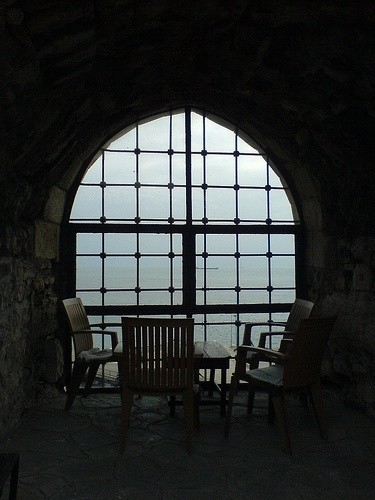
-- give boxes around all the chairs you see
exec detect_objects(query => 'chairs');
[237,298,317,425]
[54,297,138,416]
[112,315,208,462]
[227,315,337,456]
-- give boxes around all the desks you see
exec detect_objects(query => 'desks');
[112,340,231,443]
